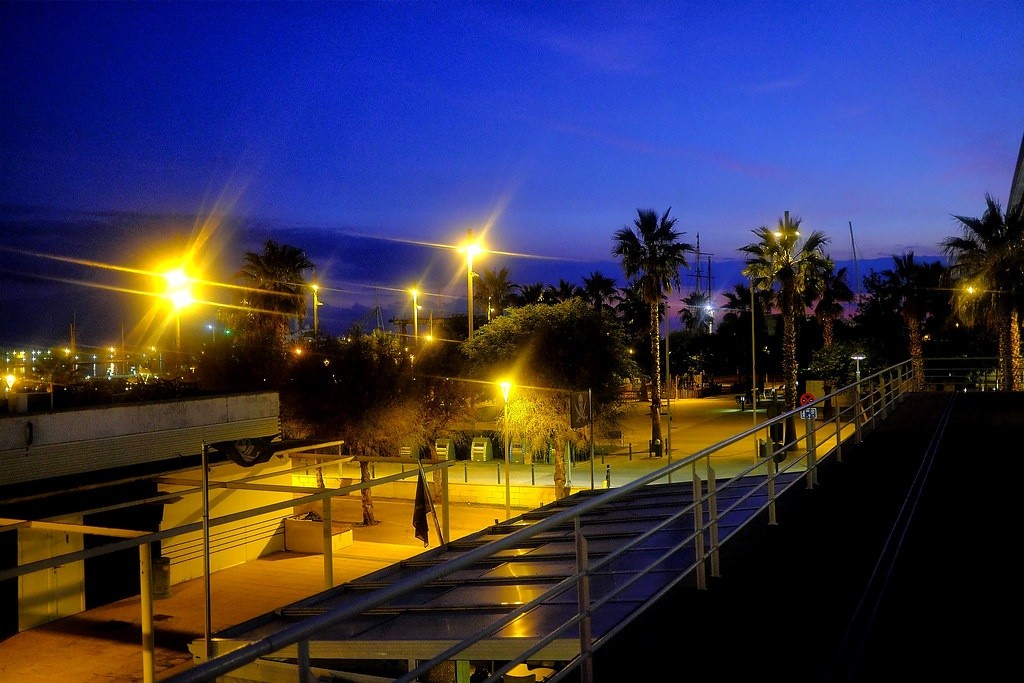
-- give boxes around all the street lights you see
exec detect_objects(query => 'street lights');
[166,270,190,376]
[309,278,322,336]
[851,350,864,445]
[408,287,419,352]
[457,228,487,345]
[495,381,514,518]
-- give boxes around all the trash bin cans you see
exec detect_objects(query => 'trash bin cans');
[400,445,420,457]
[435,438,456,460]
[759,439,767,457]
[510,438,531,464]
[549,438,570,465]
[470,437,493,462]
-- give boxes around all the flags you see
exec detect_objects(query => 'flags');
[413,472,433,549]
